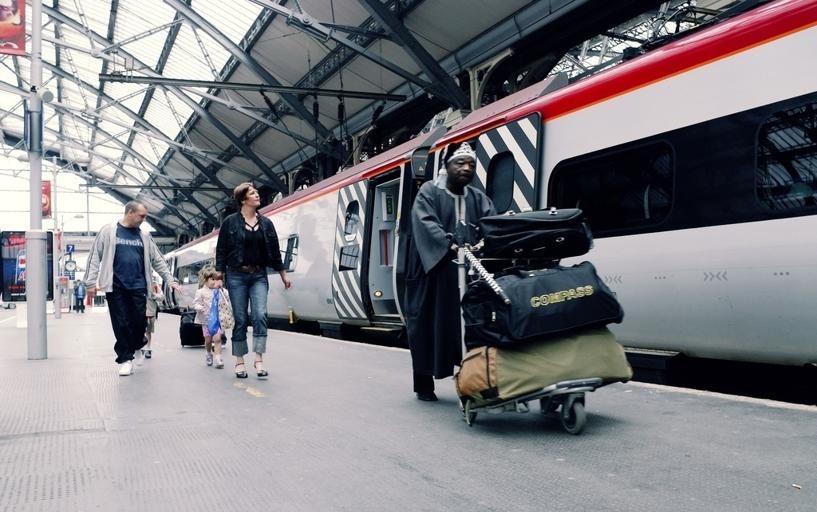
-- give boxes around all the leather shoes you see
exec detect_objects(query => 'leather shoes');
[235,363,247,378]
[254,361,268,376]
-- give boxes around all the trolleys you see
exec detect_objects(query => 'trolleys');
[450,244,602,435]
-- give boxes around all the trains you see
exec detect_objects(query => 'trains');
[149,1,813,370]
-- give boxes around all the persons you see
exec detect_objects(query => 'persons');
[191,265,234,369]
[399,142,500,403]
[139,270,166,359]
[72,278,104,314]
[82,199,185,379]
[210,182,293,379]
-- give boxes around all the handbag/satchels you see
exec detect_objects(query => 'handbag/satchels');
[457,326,633,411]
[218,288,235,329]
[460,262,623,350]
[479,209,589,260]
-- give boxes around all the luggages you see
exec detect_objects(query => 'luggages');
[180,310,205,347]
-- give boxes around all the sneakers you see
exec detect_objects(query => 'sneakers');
[118,360,134,376]
[135,343,151,366]
[207,354,224,369]
[416,392,438,401]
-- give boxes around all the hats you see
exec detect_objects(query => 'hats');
[444,140,477,164]
